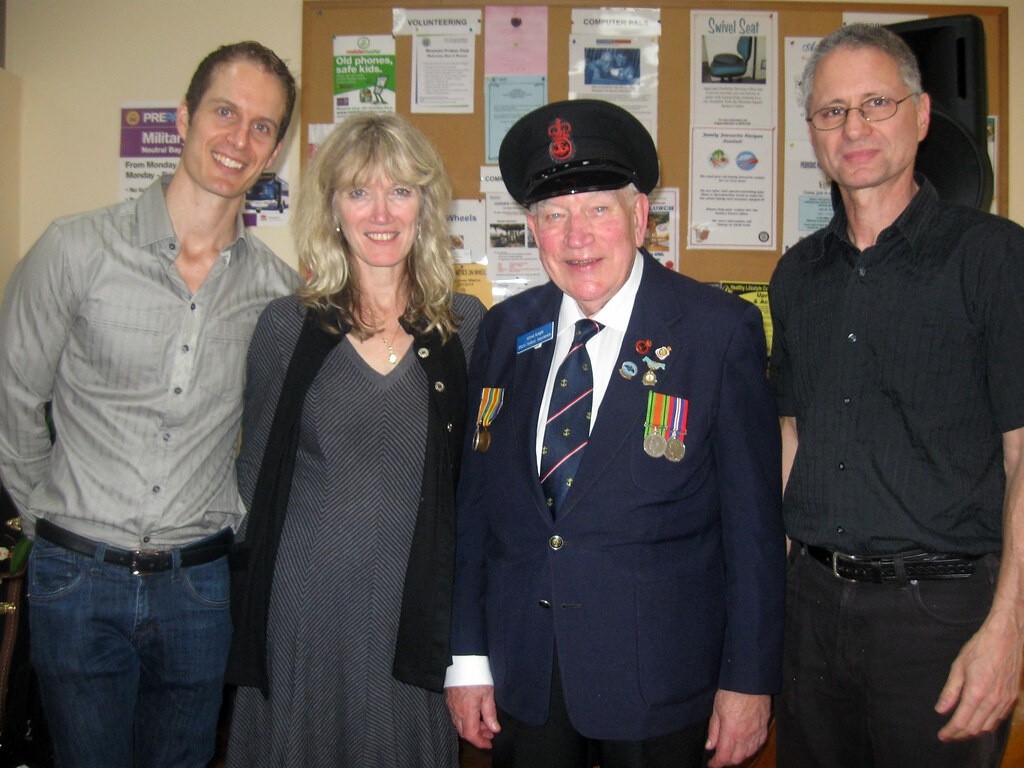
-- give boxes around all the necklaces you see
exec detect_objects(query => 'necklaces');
[379,324,402,365]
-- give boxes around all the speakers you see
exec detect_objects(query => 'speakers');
[831,14,994,217]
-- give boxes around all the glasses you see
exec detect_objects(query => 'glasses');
[806,92,921,131]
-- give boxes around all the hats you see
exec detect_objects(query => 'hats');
[498,98,660,212]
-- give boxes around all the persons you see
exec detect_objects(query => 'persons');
[0,39,309,768]
[221,112,489,768]
[441,99,787,768]
[769,22,1024,768]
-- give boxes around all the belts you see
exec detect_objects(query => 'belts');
[796,539,978,583]
[35,518,236,576]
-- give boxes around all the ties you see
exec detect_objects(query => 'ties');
[540,319,605,521]
